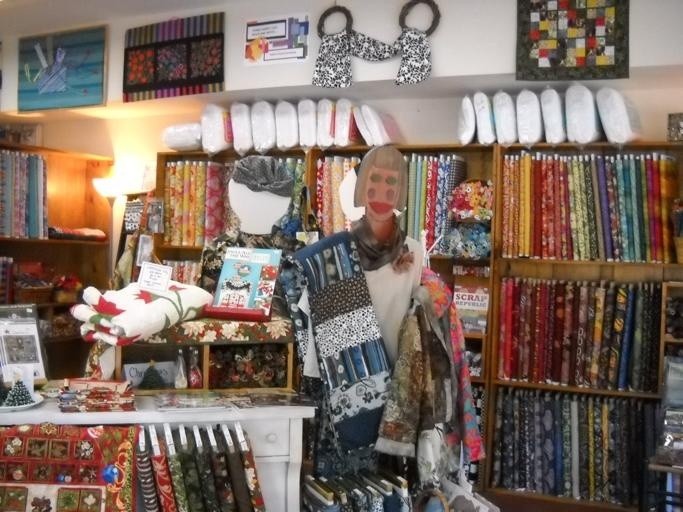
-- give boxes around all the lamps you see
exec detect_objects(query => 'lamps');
[89,177,129,295]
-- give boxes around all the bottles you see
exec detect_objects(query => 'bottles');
[189,347,203,388]
[175,348,188,389]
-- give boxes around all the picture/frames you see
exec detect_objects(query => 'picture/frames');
[514,0,629,81]
[15,23,109,115]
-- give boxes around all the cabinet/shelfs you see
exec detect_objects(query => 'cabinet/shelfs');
[0,142,115,375]
[483,146,668,510]
[304,150,495,487]
[155,149,303,312]
[0,388,318,512]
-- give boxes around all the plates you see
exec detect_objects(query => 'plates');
[0,393,45,413]
